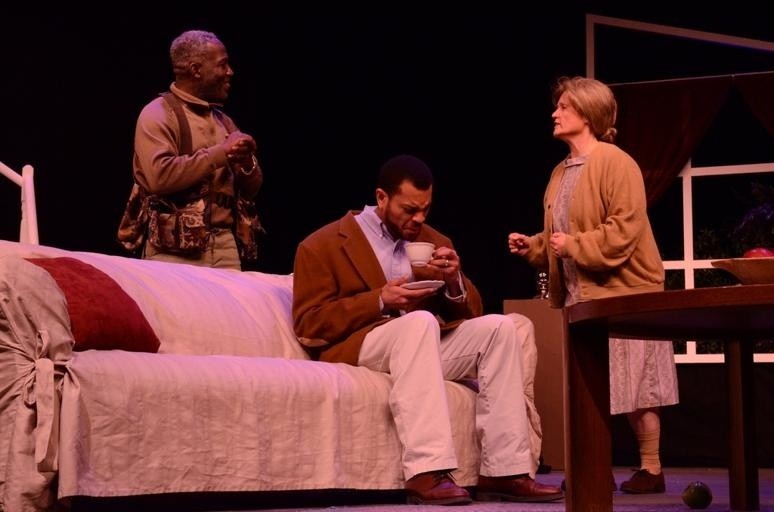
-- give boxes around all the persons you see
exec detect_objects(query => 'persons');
[508,76,679,496]
[116,31,266,273]
[291,153,565,507]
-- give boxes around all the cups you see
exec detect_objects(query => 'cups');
[404,242,437,268]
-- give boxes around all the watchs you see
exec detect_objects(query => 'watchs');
[242,153,256,174]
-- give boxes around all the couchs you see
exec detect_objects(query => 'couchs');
[0,239,542,511]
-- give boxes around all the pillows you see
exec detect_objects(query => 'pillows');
[26,256,161,354]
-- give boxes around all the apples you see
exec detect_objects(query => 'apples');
[743,248,774,259]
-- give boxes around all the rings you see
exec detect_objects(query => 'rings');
[444,259,450,268]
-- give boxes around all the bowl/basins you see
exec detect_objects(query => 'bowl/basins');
[711,258,774,286]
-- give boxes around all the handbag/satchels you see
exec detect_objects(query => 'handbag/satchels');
[236,190,267,263]
[145,181,211,255]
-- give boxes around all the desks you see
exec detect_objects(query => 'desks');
[564,283,773,512]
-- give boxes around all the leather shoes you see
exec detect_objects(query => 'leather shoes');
[403,470,474,507]
[561,471,617,492]
[476,473,564,504]
[621,469,666,495]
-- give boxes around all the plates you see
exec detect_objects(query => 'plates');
[396,280,446,293]
